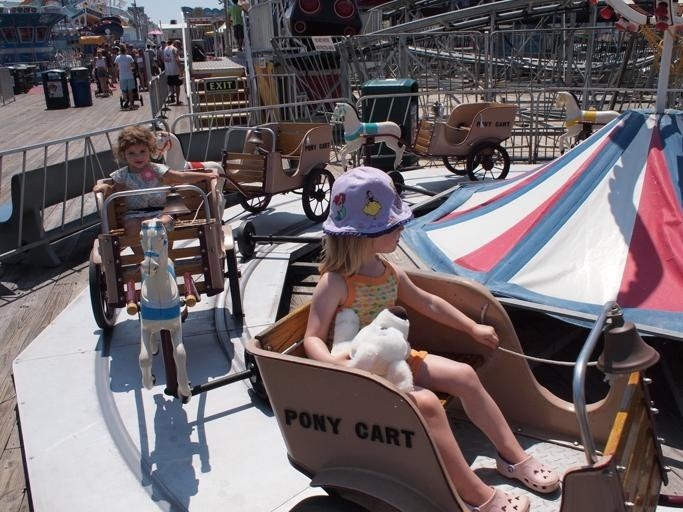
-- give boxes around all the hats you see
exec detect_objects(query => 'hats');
[321,166,414,239]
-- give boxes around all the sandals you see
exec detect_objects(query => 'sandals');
[466,486,530,512]
[496,452,560,493]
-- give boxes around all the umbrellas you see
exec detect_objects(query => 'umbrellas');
[147,29,164,35]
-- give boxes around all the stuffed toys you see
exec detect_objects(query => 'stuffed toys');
[332,306,414,391]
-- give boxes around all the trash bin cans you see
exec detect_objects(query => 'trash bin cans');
[360,78,418,170]
[67,67,92,108]
[41,69,71,110]
[5,64,39,95]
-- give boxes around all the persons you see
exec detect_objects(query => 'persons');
[227,0,248,51]
[93,126,219,260]
[302,168,560,511]
[71,36,204,111]
